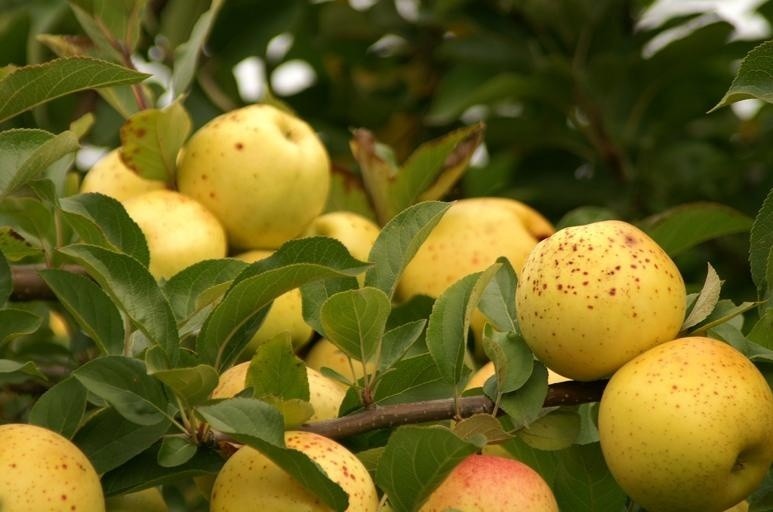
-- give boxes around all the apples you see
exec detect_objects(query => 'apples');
[0,103,773,512]
